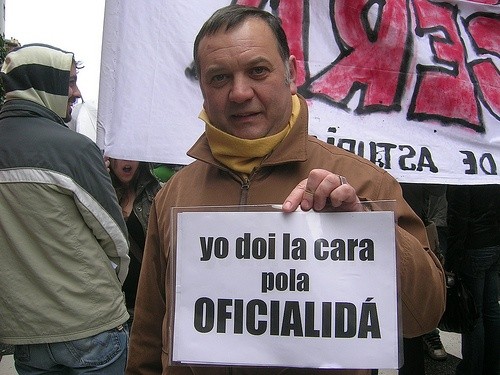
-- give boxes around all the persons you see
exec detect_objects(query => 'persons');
[1,41,128,375]
[103,155,163,334]
[398,183,500,375]
[125,5,447,372]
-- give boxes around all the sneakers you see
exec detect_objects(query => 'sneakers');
[423,330,448,360]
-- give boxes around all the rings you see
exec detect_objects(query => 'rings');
[338,174,347,185]
